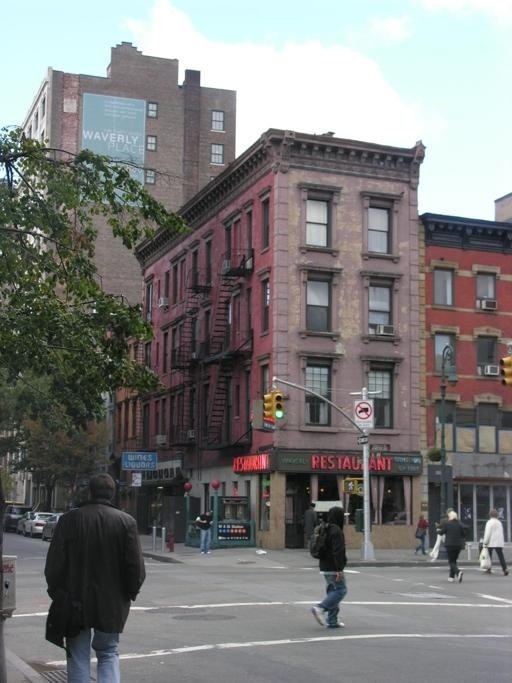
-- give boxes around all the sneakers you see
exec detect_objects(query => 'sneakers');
[200,550,212,555]
[457,570,463,583]
[447,577,455,583]
[484,568,493,575]
[312,604,328,627]
[330,622,346,628]
[504,569,509,576]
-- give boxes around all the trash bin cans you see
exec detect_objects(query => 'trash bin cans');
[468,541,480,561]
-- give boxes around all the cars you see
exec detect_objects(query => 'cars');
[386,510,408,523]
[3,499,64,543]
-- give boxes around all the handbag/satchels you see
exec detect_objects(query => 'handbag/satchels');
[414,526,426,538]
[42,576,89,654]
[479,548,493,568]
[195,520,206,528]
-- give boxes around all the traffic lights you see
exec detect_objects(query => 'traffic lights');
[262,393,273,419]
[274,392,283,418]
[345,478,356,492]
[498,354,512,387]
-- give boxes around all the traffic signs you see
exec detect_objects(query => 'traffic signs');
[357,436,370,444]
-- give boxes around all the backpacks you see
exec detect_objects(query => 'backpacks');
[309,521,332,560]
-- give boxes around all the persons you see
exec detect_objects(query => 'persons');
[435,507,454,528]
[45,472,147,681]
[414,513,430,555]
[435,510,465,583]
[197,507,214,553]
[482,508,509,576]
[302,502,319,548]
[311,505,348,628]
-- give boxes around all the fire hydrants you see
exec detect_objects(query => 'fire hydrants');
[166,526,176,552]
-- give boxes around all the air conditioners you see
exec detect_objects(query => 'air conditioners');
[376,323,397,337]
[481,298,498,311]
[220,257,234,274]
[187,429,196,439]
[483,364,500,376]
[158,297,170,308]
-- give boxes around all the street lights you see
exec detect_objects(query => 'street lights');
[431,344,458,558]
